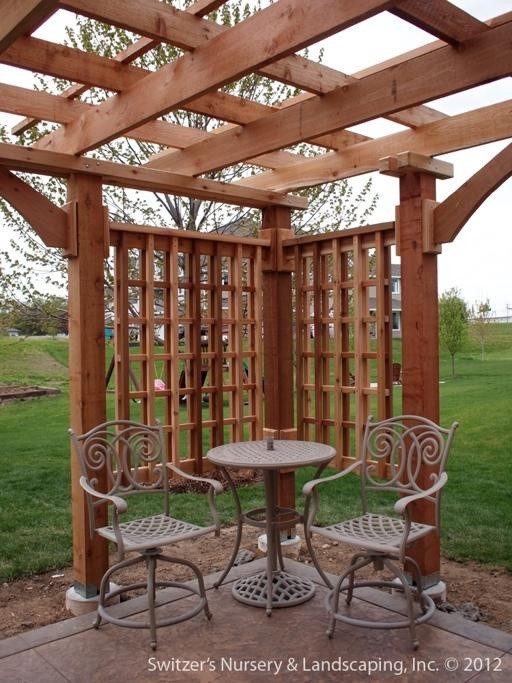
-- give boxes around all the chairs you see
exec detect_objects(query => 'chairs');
[301,415,459,653]
[67,420,226,651]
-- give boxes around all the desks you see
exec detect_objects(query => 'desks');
[207,439,336,617]
